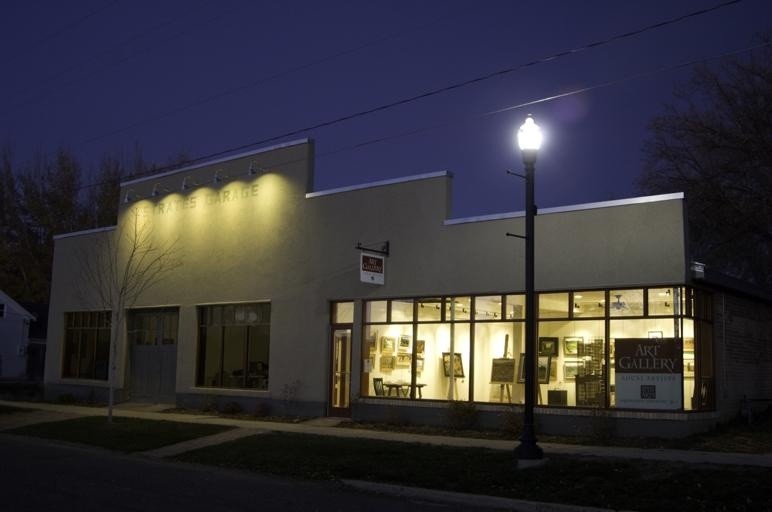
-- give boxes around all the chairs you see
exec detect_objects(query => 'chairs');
[373,377,399,397]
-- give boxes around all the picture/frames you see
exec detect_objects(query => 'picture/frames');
[517,335,615,384]
[441,351,463,377]
[491,358,515,383]
[369,326,426,373]
[648,330,695,380]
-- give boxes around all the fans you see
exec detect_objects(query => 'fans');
[593,295,636,312]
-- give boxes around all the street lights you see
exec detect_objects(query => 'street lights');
[506,111,548,463]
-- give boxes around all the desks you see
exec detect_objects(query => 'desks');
[402,383,428,399]
[383,383,403,397]
